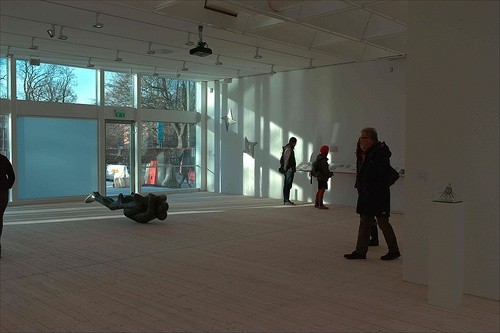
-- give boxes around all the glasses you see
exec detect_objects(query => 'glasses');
[360,136,367,140]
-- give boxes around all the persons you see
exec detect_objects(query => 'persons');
[0,154,16,236]
[344,128,401,260]
[178,147,192,188]
[354,138,379,246]
[314,145,334,210]
[278,137,297,205]
[85,191,169,223]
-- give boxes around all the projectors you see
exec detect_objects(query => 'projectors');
[189,41,212,57]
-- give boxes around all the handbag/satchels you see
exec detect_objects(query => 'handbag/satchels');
[389,165,400,187]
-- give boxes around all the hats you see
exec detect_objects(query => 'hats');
[320,145,329,154]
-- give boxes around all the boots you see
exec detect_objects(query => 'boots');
[314,199,329,210]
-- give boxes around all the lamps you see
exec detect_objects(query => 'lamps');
[5,46,13,58]
[29,14,277,77]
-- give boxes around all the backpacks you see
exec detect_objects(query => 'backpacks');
[311,157,327,177]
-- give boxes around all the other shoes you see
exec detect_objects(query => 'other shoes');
[366,239,380,247]
[284,201,295,206]
[380,252,401,260]
[343,250,367,260]
